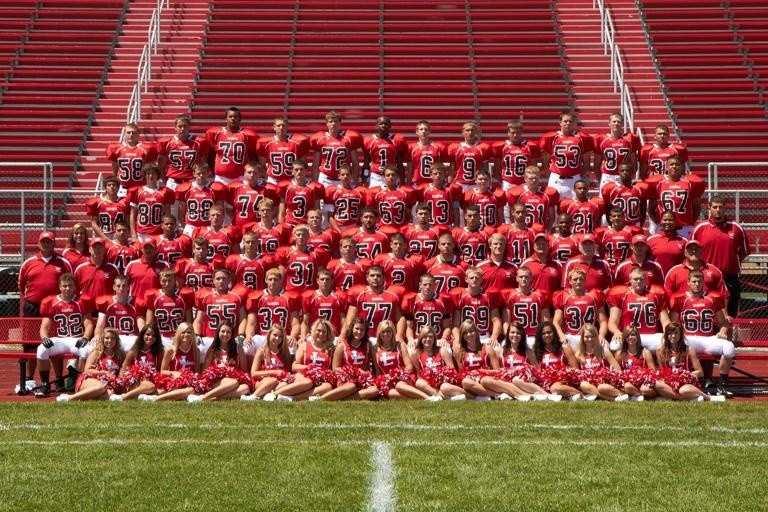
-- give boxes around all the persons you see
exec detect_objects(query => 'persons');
[15,107,750,401]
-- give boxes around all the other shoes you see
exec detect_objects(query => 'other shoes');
[425,394,645,403]
[688,379,733,402]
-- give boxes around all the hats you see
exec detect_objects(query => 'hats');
[39,231,56,242]
[534,233,703,250]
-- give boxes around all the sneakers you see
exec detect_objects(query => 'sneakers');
[308,394,322,402]
[263,390,276,401]
[241,394,260,401]
[54,378,66,392]
[56,393,69,404]
[138,393,157,403]
[277,394,294,402]
[34,381,51,396]
[110,394,125,402]
[186,394,203,403]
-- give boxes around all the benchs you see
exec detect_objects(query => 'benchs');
[0,353,768,396]
[0,0,768,259]
